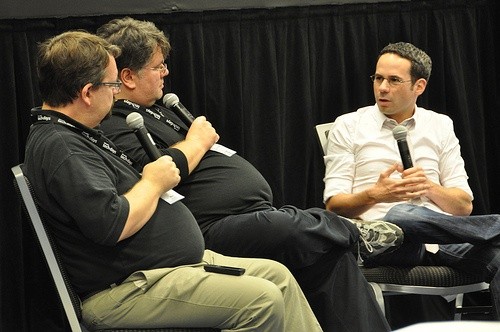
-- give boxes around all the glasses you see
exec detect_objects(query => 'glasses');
[120,62,167,74]
[81,79,122,94]
[370,74,415,88]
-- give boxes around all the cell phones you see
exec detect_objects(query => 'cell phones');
[204,265,246,276]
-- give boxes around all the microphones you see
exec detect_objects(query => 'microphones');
[162,93,195,129]
[393,125,413,171]
[127,112,161,161]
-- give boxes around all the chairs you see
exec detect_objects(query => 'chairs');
[12,164,230,332]
[315,123,493,321]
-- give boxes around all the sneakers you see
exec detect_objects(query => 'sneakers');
[338,215,404,266]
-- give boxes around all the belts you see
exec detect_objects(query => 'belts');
[80,280,122,301]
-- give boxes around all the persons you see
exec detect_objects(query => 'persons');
[96,17,405,332]
[324,42,500,317]
[24,32,323,332]
